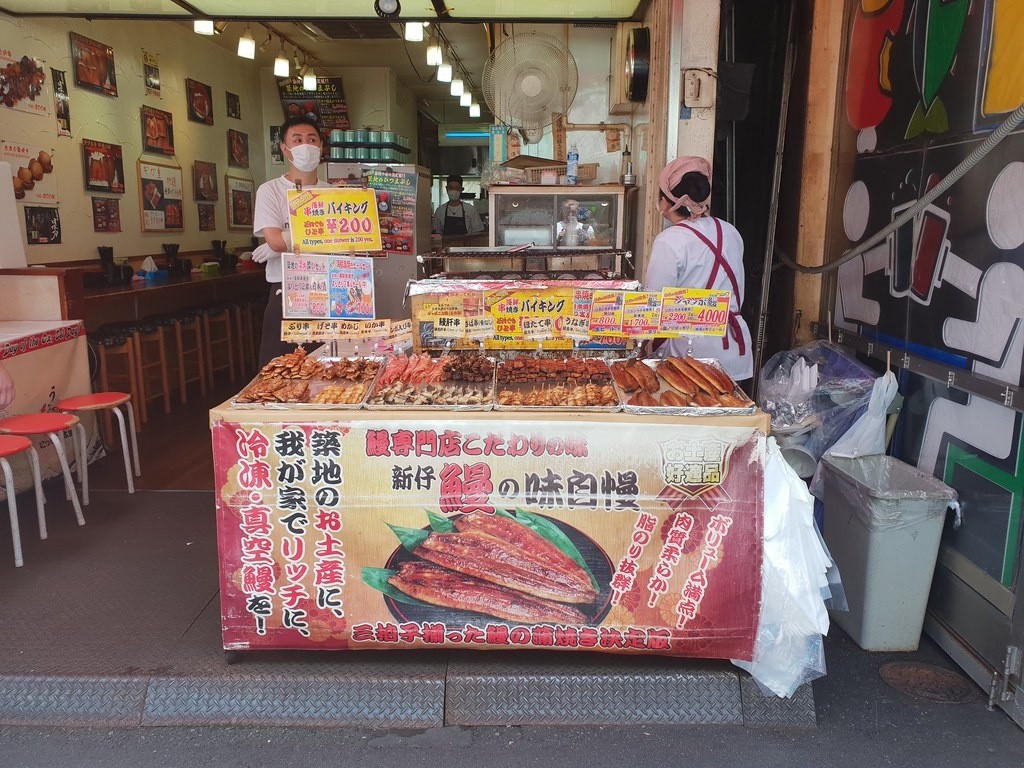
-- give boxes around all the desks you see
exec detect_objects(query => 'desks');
[56,392,142,501]
[83,265,266,302]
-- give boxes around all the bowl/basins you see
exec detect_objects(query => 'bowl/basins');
[780,445,817,478]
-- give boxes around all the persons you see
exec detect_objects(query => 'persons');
[636,155,753,397]
[552,200,596,245]
[432,174,486,235]
[252,117,339,371]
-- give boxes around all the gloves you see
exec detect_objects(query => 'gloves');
[251,242,281,264]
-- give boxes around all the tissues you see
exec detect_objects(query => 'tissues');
[201,262,220,272]
[140,255,168,279]
[239,251,255,267]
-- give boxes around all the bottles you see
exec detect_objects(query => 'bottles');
[566,143,579,186]
[621,144,632,174]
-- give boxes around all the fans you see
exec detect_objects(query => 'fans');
[480,30,579,145]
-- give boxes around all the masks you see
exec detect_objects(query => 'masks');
[280,144,322,173]
[448,190,460,200]
[656,197,669,215]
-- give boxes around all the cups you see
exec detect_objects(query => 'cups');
[102,263,114,277]
[114,264,134,282]
[176,259,194,273]
[167,257,177,265]
[224,255,238,267]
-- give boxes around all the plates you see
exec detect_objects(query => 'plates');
[384,509,616,630]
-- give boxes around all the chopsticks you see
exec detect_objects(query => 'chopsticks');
[212,240,226,252]
[97,246,113,263]
[162,244,179,256]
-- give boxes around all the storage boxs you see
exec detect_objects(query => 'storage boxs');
[500,228,551,245]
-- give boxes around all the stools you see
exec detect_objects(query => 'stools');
[0,435,48,568]
[85,298,264,447]
[0,412,89,526]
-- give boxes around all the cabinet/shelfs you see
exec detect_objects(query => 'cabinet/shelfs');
[606,21,642,115]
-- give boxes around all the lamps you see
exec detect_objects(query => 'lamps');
[237,21,255,60]
[273,37,289,77]
[258,28,272,53]
[214,20,229,34]
[297,52,317,91]
[436,43,452,83]
[293,46,302,70]
[193,20,213,36]
[405,22,424,42]
[450,60,481,118]
[426,23,442,66]
[373,0,401,18]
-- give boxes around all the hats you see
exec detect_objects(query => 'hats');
[446,174,463,185]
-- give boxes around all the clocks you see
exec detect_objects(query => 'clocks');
[624,26,649,102]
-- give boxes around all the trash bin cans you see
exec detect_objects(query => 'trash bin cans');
[820,454,957,651]
